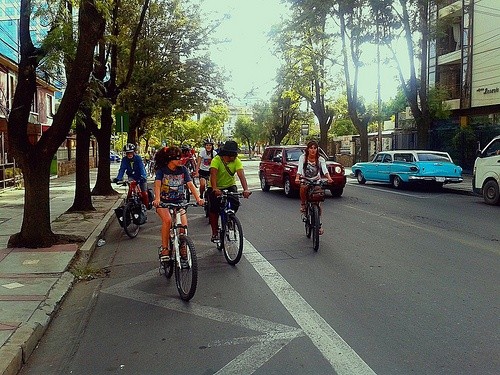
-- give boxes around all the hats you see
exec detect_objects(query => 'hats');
[222,140,241,153]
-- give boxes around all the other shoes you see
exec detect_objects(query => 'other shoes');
[318,227,324,235]
[300,212,307,221]
[179,245,187,259]
[161,247,170,262]
[227,222,234,231]
[210,235,218,241]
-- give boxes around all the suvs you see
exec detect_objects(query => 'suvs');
[258,144,347,198]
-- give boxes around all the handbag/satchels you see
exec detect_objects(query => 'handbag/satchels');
[309,184,325,203]
[114,205,131,227]
[132,203,147,225]
[147,188,154,210]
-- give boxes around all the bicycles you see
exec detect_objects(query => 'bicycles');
[153,200,208,301]
[144,158,155,178]
[300,176,332,251]
[212,192,252,264]
[111,178,149,237]
[184,173,196,208]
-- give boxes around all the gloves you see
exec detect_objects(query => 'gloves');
[140,177,146,183]
[113,178,119,183]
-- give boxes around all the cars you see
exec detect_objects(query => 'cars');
[110,151,120,163]
[351,150,465,190]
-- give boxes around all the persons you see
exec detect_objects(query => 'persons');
[152,145,205,269]
[195,141,218,203]
[295,140,334,235]
[113,143,149,209]
[207,141,251,242]
[143,141,221,178]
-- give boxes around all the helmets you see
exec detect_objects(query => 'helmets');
[204,138,214,144]
[123,143,135,151]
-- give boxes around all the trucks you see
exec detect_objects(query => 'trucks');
[472,135,500,205]
